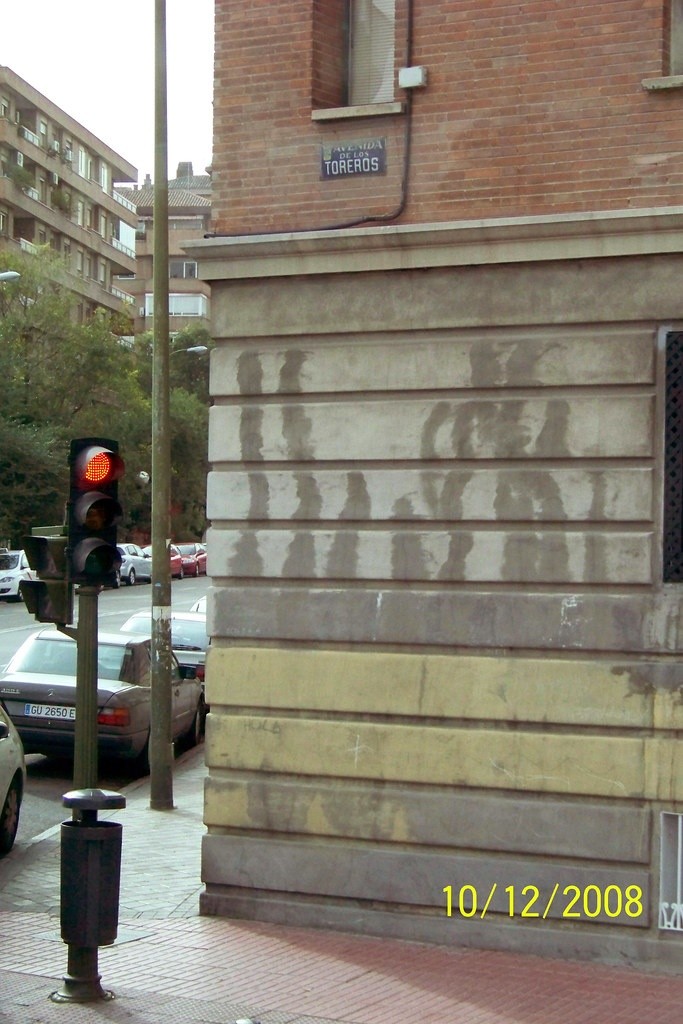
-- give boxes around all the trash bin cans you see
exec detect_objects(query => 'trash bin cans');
[58,788,127,950]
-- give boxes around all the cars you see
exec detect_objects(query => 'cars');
[0,548,39,603]
[190,596,207,615]
[120,608,206,714]
[0,697,27,858]
[102,542,152,590]
[0,626,207,776]
[172,541,207,578]
[140,542,185,580]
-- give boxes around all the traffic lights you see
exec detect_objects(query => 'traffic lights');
[18,524,73,626]
[66,436,122,588]
